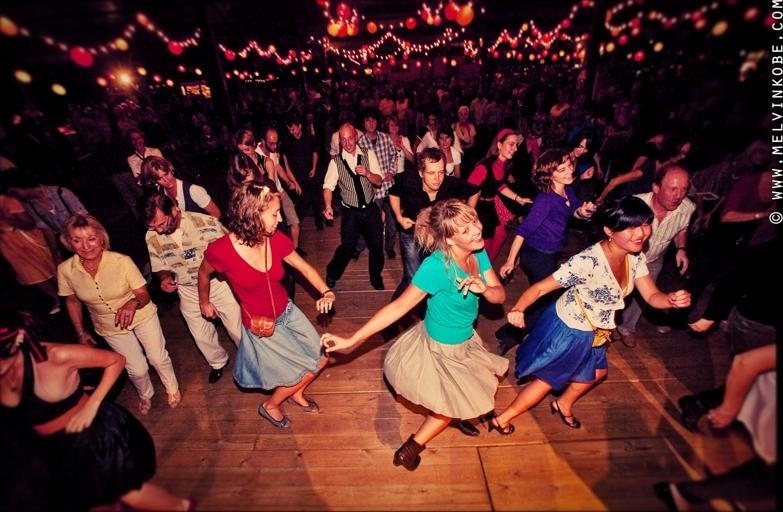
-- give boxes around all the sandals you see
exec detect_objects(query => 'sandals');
[168,391,181,408]
[139,400,151,414]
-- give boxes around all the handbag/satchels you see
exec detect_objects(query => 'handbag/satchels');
[242,238,277,337]
[574,256,630,348]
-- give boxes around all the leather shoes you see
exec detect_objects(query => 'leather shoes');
[488,418,515,435]
[452,420,481,437]
[619,326,635,347]
[388,250,396,258]
[550,401,581,428]
[288,395,319,413]
[393,435,425,470]
[258,404,293,429]
[373,278,384,289]
[653,481,678,511]
[209,359,229,383]
[326,272,335,287]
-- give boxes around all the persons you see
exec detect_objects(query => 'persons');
[468,64,536,134]
[312,75,358,129]
[126,129,165,186]
[656,169,783,335]
[275,140,302,196]
[319,200,505,472]
[322,124,384,290]
[416,114,438,145]
[384,116,414,186]
[141,155,222,218]
[677,238,783,433]
[0,193,61,315]
[162,90,236,159]
[197,180,335,428]
[494,149,598,357]
[488,194,691,437]
[121,88,164,131]
[0,93,125,185]
[0,167,90,260]
[0,326,196,512]
[138,191,244,384]
[466,129,535,263]
[349,112,397,262]
[652,343,783,512]
[435,126,461,178]
[357,66,469,116]
[416,118,464,159]
[387,148,481,302]
[280,119,324,232]
[534,61,783,180]
[233,82,287,136]
[56,214,181,415]
[233,129,276,188]
[612,163,696,348]
[451,106,477,148]
[283,84,312,118]
[304,112,315,137]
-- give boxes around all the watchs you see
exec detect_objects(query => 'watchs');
[676,247,686,250]
[130,297,141,310]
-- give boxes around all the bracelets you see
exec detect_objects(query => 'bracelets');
[322,289,333,296]
[78,331,88,338]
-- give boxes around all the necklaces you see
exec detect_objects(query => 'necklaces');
[5,364,19,393]
